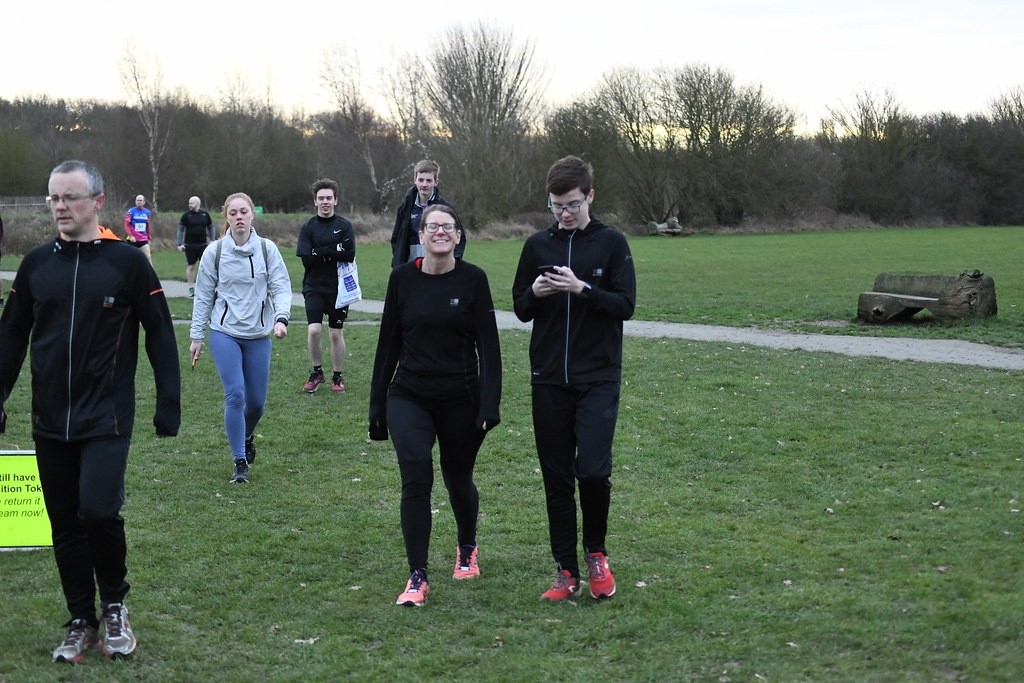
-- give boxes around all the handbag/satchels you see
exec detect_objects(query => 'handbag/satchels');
[334,243,362,308]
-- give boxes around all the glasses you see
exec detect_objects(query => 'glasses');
[547,196,587,214]
[424,222,456,233]
[45,192,97,207]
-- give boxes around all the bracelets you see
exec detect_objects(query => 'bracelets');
[578,282,591,299]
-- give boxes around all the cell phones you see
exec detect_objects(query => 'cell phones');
[192,353,196,370]
[538,265,558,281]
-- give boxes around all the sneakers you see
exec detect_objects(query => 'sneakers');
[585,547,617,599]
[230,458,249,483]
[539,563,581,602]
[245,435,255,464]
[397,570,430,608]
[453,543,480,580]
[188,287,194,297]
[53,618,102,663]
[330,377,345,394]
[99,602,136,657]
[303,369,324,392]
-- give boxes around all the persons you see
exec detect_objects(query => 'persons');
[369,205,502,607]
[124,195,153,266]
[177,196,215,296]
[390,160,466,268]
[0,160,181,662]
[190,192,292,484]
[511,155,636,600]
[296,179,356,393]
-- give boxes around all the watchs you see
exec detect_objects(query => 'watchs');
[277,318,288,326]
